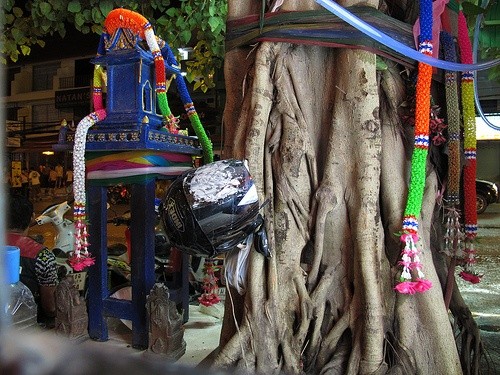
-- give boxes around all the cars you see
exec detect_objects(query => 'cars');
[477,180,498,215]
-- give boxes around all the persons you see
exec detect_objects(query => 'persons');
[0,162,74,203]
[5,195,61,331]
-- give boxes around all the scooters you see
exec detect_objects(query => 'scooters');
[27,199,75,274]
[108,208,171,285]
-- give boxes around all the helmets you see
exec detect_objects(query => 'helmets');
[160,156,275,260]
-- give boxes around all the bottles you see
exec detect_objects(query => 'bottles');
[4,246,37,330]
